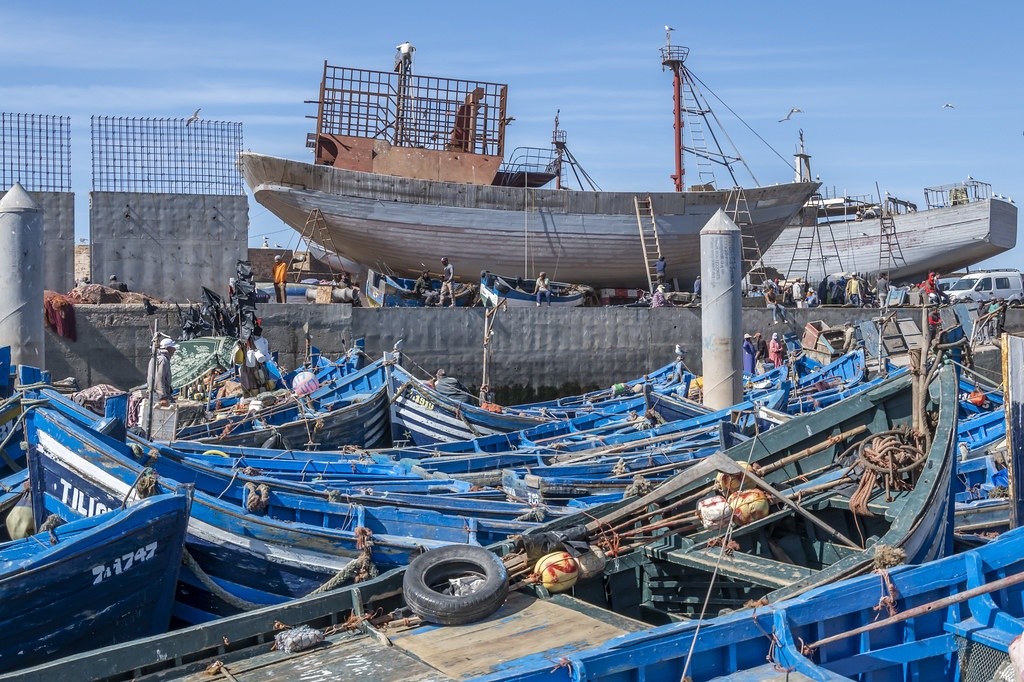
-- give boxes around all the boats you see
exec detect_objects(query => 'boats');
[478,271,594,307]
[737,128,1018,288]
[0,299,1024,682]
[364,267,478,308]
[236,33,823,291]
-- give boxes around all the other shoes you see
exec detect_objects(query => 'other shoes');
[449,304,456,307]
[774,321,778,324]
[537,303,540,307]
[435,302,443,307]
[783,320,789,323]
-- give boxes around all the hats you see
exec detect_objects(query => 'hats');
[754,333,761,338]
[852,273,857,275]
[743,333,752,338]
[772,332,779,339]
[438,369,446,376]
[161,338,180,349]
[658,285,667,289]
[842,322,851,326]
[990,297,997,300]
[976,299,983,302]
[998,297,1004,301]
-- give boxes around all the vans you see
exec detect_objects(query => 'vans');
[934,270,1024,313]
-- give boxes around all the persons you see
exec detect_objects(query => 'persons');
[765,285,789,324]
[976,297,1007,345]
[532,272,552,307]
[846,272,863,304]
[842,321,857,353]
[414,257,456,308]
[743,333,783,375]
[773,278,818,307]
[819,277,829,304]
[691,276,701,303]
[271,255,287,303]
[878,273,889,307]
[653,256,666,285]
[434,368,472,404]
[396,41,416,74]
[928,306,942,340]
[148,338,180,407]
[830,280,843,303]
[652,285,668,307]
[925,273,949,304]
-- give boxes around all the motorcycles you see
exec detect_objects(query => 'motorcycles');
[227,257,259,305]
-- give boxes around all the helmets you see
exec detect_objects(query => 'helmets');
[441,257,448,261]
